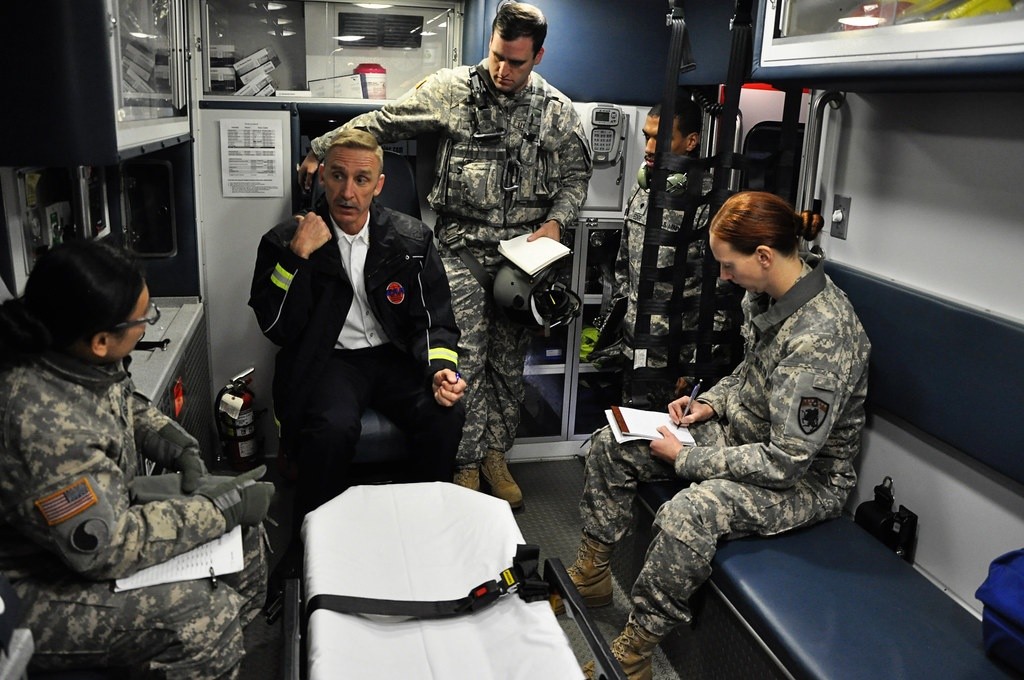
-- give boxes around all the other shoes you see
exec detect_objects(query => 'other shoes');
[262,552,296,626]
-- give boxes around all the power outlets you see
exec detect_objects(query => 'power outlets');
[830,193,851,240]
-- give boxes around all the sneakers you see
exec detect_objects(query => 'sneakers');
[454,467,479,493]
[480,446,523,508]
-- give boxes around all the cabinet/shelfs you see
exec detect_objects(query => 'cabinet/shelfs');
[751,0,1024,91]
[0,0,193,164]
[503,211,625,458]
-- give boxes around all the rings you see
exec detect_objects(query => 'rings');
[452,384,454,392]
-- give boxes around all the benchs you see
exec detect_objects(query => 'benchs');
[631,262,1024,680]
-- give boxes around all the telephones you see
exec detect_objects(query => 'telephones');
[586,104,627,167]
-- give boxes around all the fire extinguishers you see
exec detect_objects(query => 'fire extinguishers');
[214,367,265,472]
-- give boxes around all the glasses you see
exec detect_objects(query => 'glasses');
[104,302,160,334]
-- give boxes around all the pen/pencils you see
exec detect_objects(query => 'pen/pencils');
[677,379,703,430]
[209,566,218,589]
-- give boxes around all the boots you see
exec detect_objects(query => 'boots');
[581,611,663,680]
[549,533,613,617]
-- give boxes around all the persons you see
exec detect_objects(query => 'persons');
[0,237,274,680]
[247,131,466,578]
[551,192,871,680]
[300,3,594,502]
[586,103,727,439]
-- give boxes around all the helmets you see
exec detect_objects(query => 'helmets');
[492,258,581,338]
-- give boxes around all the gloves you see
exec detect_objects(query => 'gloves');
[175,445,209,494]
[205,463,277,533]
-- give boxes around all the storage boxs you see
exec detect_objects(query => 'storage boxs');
[306,61,387,100]
[235,72,274,96]
[255,83,280,97]
[275,89,312,97]
[210,68,242,93]
[240,56,282,86]
[210,45,243,67]
[232,45,278,77]
[975,546,1024,680]
